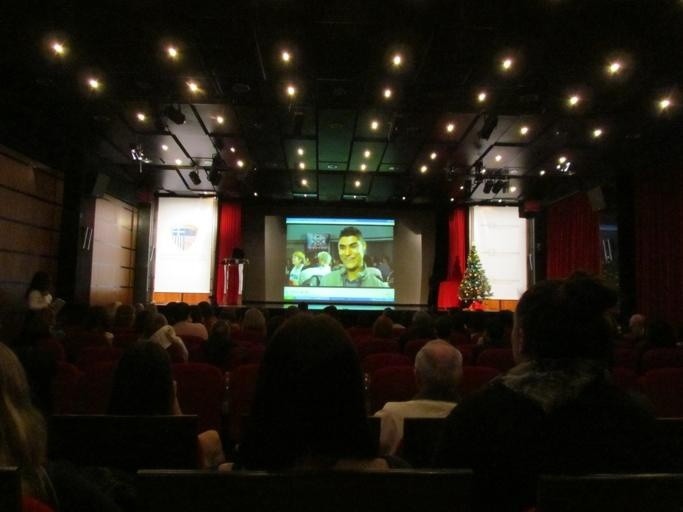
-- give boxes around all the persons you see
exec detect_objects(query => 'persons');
[284,226,393,289]
[0,340,64,511]
[14,265,679,477]
